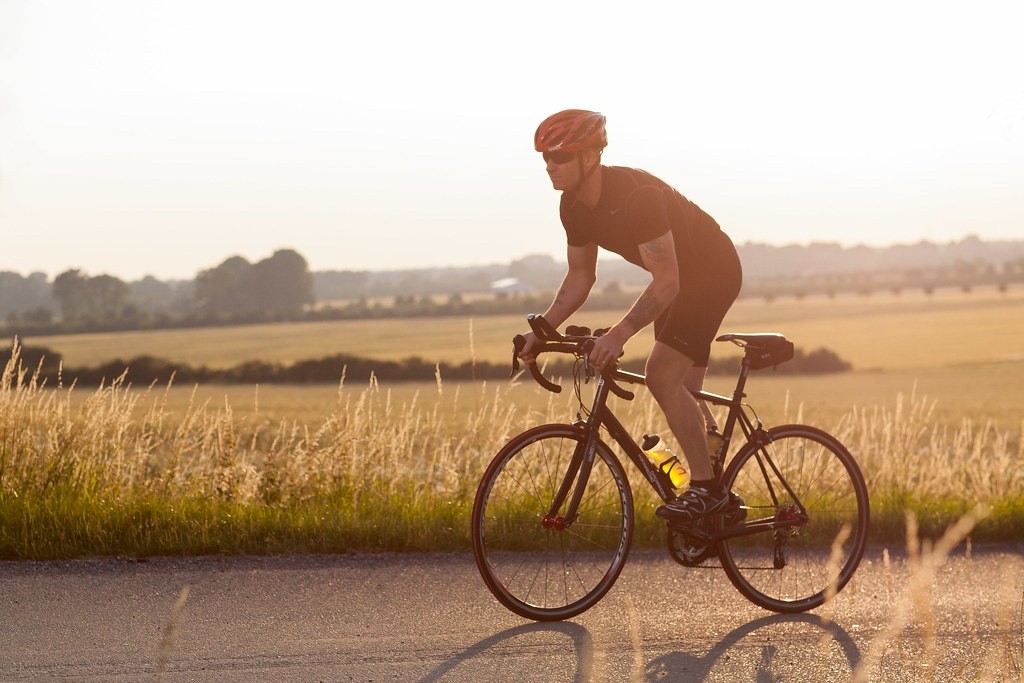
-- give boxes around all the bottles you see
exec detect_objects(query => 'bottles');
[708,426,723,467]
[642,434,689,488]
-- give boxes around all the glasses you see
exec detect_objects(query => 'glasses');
[541,149,585,165]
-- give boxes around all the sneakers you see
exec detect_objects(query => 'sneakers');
[655,478,730,521]
[690,491,748,532]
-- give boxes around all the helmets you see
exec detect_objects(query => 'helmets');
[534,109,609,155]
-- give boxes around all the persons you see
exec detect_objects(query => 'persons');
[512,109,748,530]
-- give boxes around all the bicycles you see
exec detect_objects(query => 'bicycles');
[469,313,872,621]
